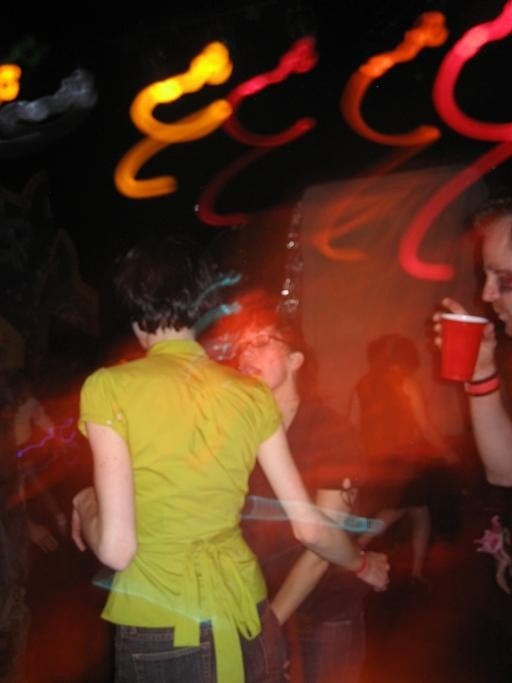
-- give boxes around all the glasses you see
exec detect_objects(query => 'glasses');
[235,334,293,355]
[469,263,511,293]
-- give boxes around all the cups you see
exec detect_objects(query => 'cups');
[439,313,491,383]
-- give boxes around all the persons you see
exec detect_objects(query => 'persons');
[0,202,511,681]
